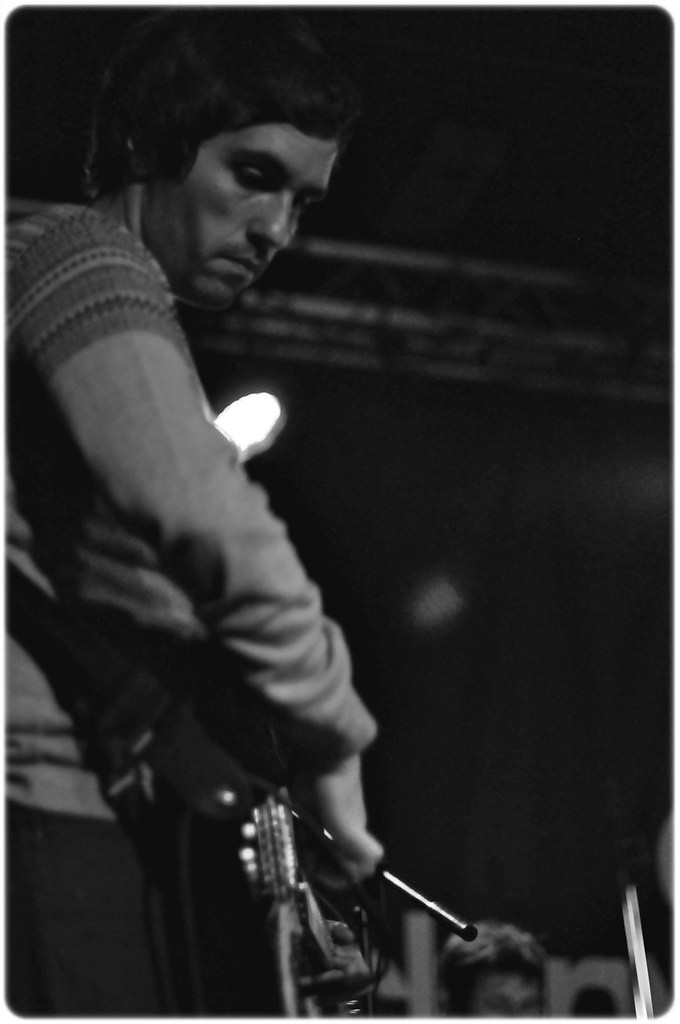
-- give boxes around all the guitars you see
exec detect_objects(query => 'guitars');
[55,498,284,828]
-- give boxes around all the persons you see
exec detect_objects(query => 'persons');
[10,11,387,1015]
[436,917,549,1017]
